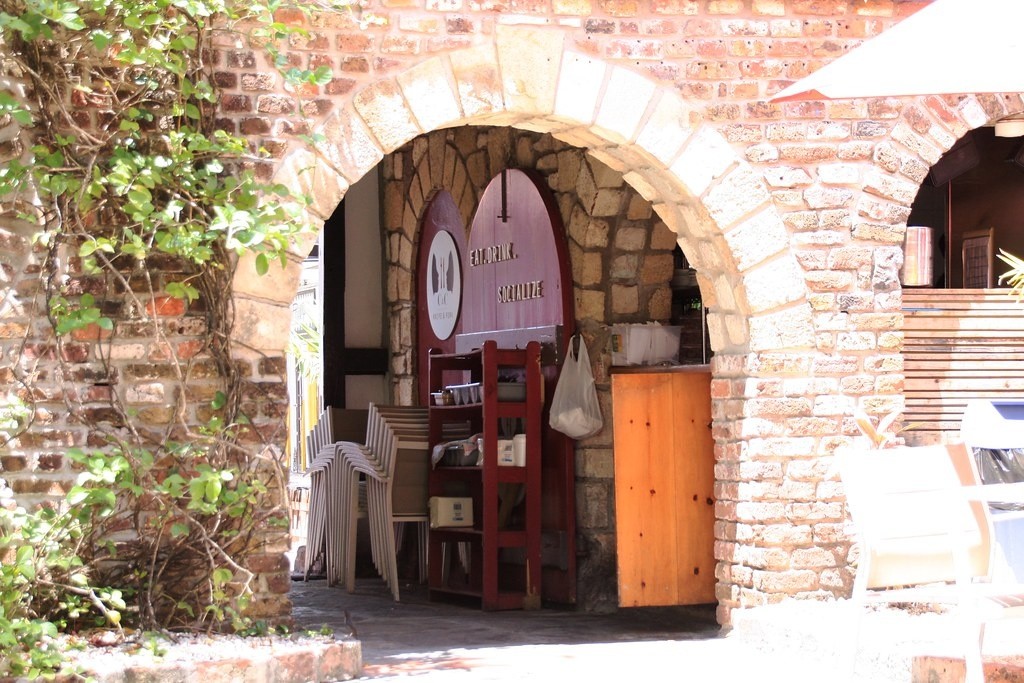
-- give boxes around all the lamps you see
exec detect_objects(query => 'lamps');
[995,119,1024,138]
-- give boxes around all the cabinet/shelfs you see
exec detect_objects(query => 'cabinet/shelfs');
[424,339,539,611]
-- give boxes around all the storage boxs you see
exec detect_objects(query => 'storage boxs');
[610,325,684,365]
[444,447,479,466]
[432,392,454,405]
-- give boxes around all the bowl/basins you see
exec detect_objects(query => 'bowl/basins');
[479,382,526,403]
[442,446,479,466]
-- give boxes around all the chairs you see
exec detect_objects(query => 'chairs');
[303,402,468,603]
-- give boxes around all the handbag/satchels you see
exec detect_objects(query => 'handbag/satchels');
[549,334,603,440]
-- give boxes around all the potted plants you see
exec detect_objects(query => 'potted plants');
[477,373,526,402]
[539,343,561,377]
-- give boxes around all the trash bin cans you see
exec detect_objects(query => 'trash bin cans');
[959,398,1024,593]
